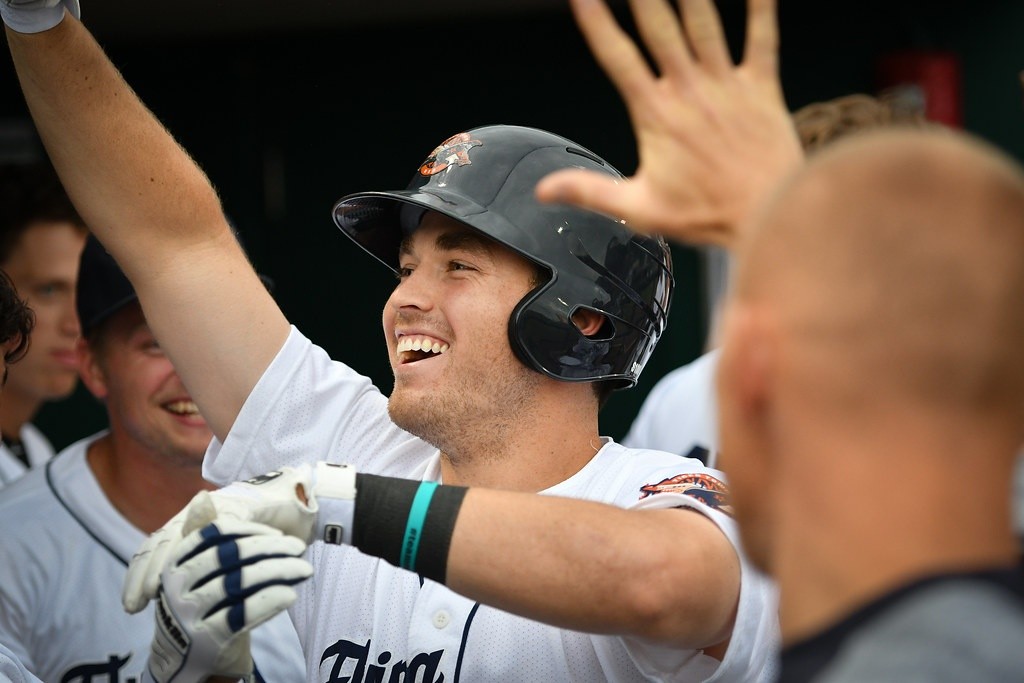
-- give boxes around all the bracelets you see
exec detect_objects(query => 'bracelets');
[401,479,438,573]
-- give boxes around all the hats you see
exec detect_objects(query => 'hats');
[75,188,274,335]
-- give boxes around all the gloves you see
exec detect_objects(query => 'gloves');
[122,460,356,615]
[140,519,314,683]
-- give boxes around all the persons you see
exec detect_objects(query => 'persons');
[1,161,91,491]
[535,0,1023,683]
[1,236,222,683]
[0,0,781,683]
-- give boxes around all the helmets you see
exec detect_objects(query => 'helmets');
[331,124,673,393]
